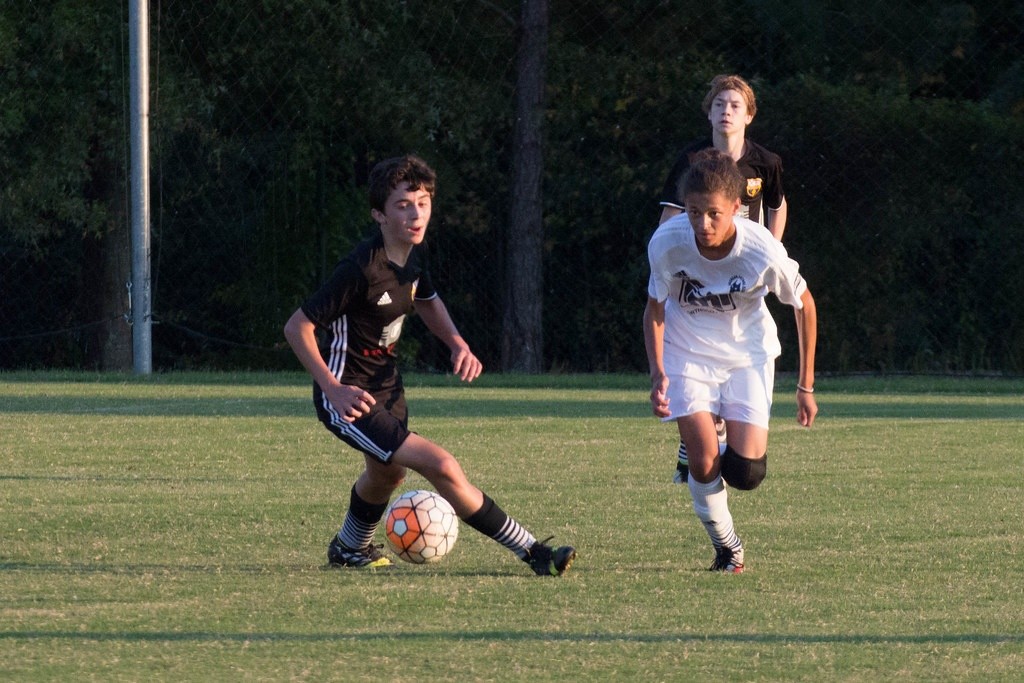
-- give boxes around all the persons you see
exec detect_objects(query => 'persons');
[656,73,787,482]
[283,156,576,575]
[644,151,818,571]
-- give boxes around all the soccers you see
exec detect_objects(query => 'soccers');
[384,487,459,564]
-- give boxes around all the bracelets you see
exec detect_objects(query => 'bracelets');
[797,384,815,393]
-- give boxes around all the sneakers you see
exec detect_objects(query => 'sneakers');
[326,535,390,567]
[711,543,746,573]
[525,533,579,579]
